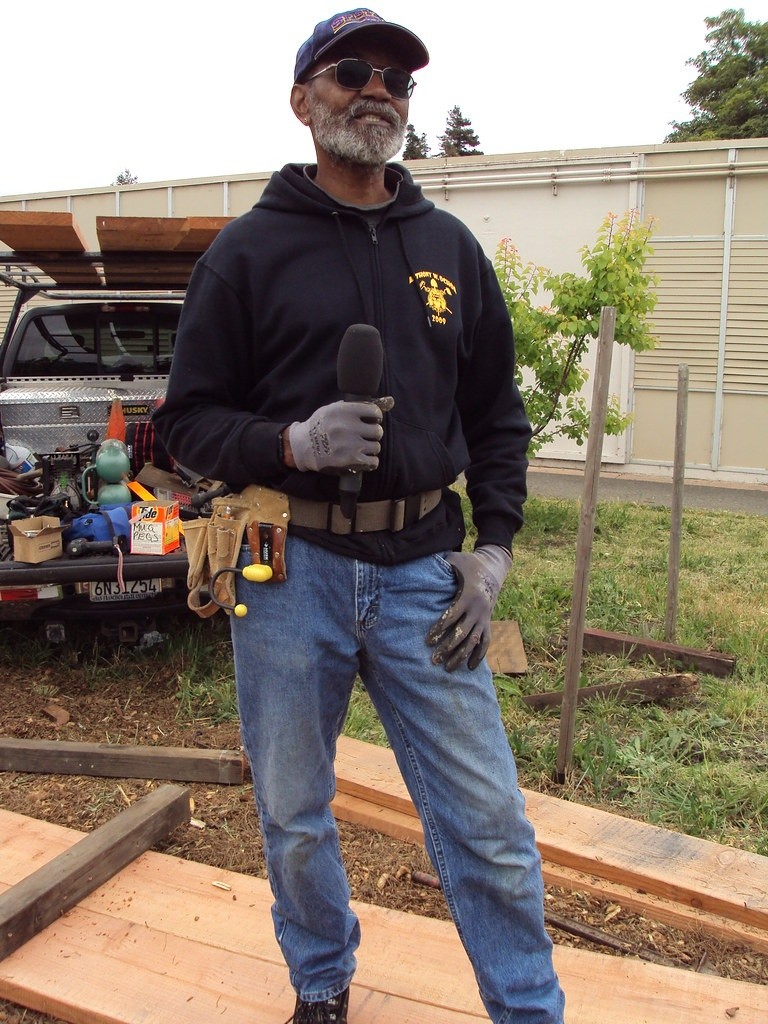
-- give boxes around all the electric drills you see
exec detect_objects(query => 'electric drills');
[67,534,127,556]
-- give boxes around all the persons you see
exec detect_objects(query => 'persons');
[154,9,567,1024]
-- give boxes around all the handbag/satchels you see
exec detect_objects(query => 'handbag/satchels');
[72,506,131,541]
[6,493,74,551]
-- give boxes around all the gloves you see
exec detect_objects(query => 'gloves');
[426,544,513,673]
[289,396,395,473]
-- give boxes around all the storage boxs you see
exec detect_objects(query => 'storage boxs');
[130,498,181,556]
[8,515,71,564]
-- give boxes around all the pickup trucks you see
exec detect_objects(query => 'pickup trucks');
[0,249,211,643]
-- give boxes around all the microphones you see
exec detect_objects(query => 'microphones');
[333,324,384,519]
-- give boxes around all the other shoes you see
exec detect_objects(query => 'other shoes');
[284,985,350,1024]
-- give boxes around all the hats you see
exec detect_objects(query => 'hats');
[294,8,429,84]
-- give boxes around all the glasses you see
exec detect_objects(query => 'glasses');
[309,57,417,101]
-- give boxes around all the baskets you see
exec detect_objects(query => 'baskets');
[173,492,192,506]
